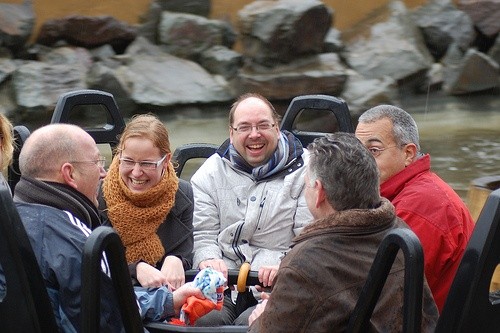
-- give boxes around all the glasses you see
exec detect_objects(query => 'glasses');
[119,155,167,171]
[68,156,106,168]
[230,121,277,133]
[369,143,409,157]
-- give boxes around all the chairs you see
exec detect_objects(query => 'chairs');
[0,89,499,333]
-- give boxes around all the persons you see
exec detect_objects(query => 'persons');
[354,104,476,317]
[249,132,441,333]
[189,92,316,326]
[94,114,196,292]
[13,123,207,333]
[0,113,14,199]
